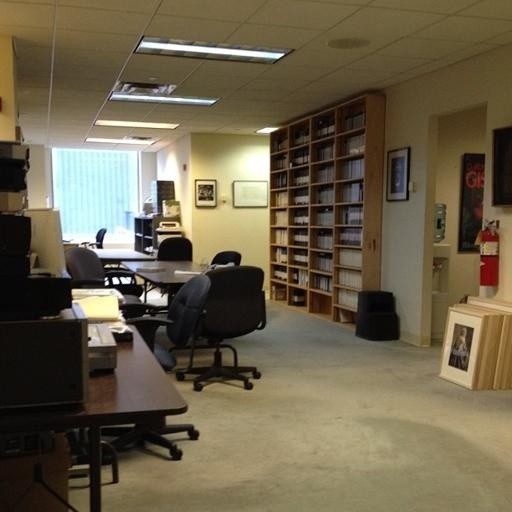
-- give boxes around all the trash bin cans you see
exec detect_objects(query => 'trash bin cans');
[355,289,399,341]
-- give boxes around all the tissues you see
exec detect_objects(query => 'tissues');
[109,323,134,342]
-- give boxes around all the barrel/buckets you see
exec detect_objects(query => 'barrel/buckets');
[433,202,448,243]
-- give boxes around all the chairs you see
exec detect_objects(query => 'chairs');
[166,265,266,391]
[85,316,200,461]
[157,239,241,269]
[81,229,107,249]
[65,247,147,320]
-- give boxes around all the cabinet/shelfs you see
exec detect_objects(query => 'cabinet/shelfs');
[134,216,157,255]
[269,93,385,322]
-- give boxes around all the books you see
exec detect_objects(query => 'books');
[271,113,366,323]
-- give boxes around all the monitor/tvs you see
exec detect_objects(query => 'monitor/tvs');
[492,124,512,208]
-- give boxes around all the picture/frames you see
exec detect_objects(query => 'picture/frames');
[232,180,268,208]
[195,179,217,207]
[437,295,512,390]
[385,146,409,202]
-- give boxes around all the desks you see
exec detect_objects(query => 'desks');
[121,259,232,325]
[42,280,189,511]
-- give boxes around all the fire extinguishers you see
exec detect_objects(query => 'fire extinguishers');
[475,218,500,287]
